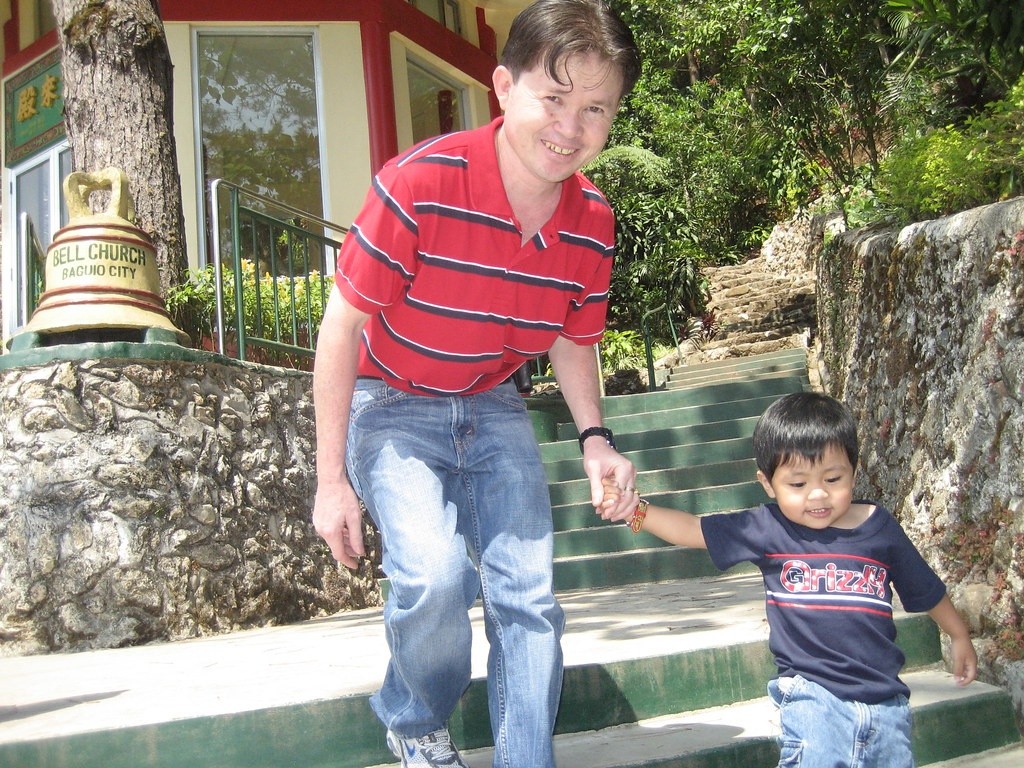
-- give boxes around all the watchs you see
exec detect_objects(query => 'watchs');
[577,427,618,454]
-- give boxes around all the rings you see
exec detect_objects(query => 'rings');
[624,486,640,497]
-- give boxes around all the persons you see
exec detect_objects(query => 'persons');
[306,1,640,768]
[593,392,981,768]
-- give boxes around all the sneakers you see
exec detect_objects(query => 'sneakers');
[385,727,470,768]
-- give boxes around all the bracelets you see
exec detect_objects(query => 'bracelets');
[626,499,649,533]
